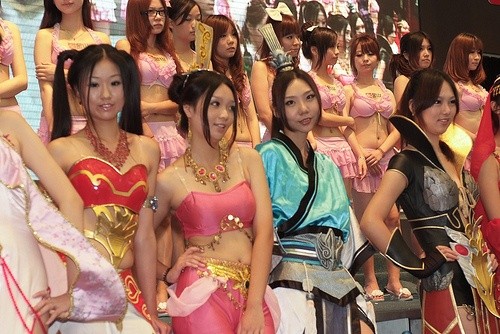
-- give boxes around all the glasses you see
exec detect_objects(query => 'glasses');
[139,9,167,17]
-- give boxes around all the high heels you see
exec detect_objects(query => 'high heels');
[384,287,413,301]
[363,284,384,301]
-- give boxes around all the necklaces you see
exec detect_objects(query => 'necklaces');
[463,81,485,94]
[174,50,194,68]
[183,143,230,192]
[316,73,335,90]
[336,55,351,74]
[356,81,381,99]
[85,120,130,172]
[60,26,86,41]
[143,50,167,63]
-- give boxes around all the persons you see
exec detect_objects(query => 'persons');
[203,14,261,150]
[153,69,275,334]
[360,69,499,334]
[254,68,376,334]
[302,27,367,209]
[114,0,190,174]
[468,75,500,333]
[88,0,412,85]
[341,34,414,301]
[390,30,436,152]
[33,0,111,144]
[248,2,317,151]
[0,107,86,334]
[164,0,213,142]
[0,0,28,115]
[442,34,490,135]
[46,44,173,334]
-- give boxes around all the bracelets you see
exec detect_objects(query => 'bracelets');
[163,266,173,286]
